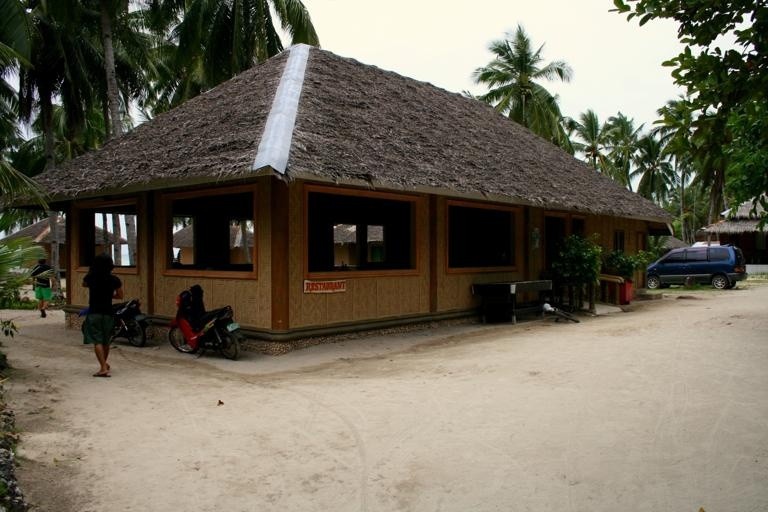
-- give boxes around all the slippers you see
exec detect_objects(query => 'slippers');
[94,363,112,378]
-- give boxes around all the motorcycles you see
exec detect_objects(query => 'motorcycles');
[166,288,242,363]
[77,292,148,348]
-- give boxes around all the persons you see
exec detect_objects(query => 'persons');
[79,252,124,378]
[30,259,56,319]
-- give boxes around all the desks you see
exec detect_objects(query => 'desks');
[469,279,553,324]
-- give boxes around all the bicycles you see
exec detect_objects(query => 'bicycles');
[541,299,580,326]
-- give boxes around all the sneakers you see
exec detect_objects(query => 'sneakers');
[40,306,46,317]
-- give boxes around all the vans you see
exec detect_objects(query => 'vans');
[644,243,747,292]
